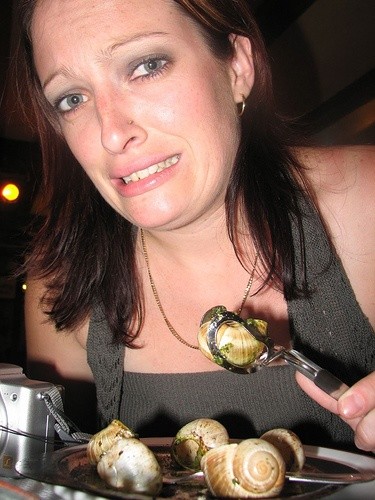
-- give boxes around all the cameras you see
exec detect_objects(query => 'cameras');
[0,363,67,479]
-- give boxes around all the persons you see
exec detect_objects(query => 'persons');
[0,0,375,454]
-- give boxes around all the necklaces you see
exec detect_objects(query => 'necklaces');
[139,227,260,350]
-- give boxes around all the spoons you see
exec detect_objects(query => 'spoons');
[163,470,375,486]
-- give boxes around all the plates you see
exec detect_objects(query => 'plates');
[15,438,375,500]
